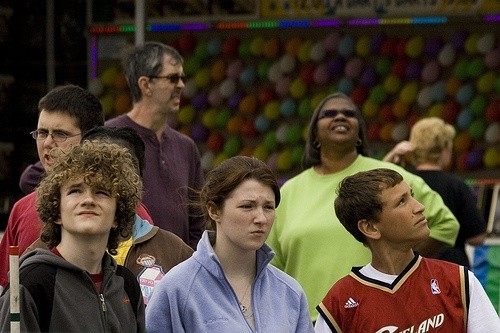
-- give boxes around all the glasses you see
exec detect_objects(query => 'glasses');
[145,72,186,83]
[29,127,87,143]
[317,109,358,119]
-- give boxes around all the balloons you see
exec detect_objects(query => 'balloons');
[86,28,499,180]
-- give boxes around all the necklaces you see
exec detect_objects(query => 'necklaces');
[236,274,257,314]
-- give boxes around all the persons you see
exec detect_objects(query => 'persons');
[139,154,315,333]
[0,137,148,333]
[18,123,197,308]
[258,89,462,333]
[310,166,500,333]
[17,39,209,259]
[0,84,155,297]
[379,114,490,295]
